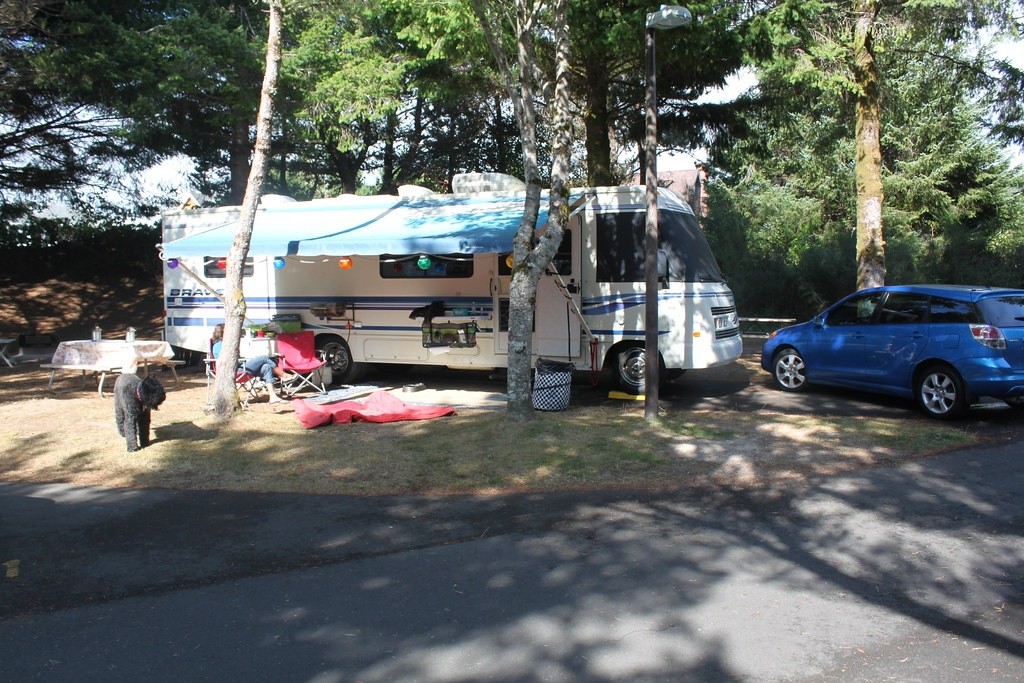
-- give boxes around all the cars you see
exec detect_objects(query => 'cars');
[761,284,1023,424]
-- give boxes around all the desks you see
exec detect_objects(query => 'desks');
[51,340,176,387]
[0,339,17,367]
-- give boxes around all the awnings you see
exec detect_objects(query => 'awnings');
[153,186,599,351]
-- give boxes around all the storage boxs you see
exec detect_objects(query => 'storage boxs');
[249,338,276,357]
[533,357,575,411]
[270,313,302,333]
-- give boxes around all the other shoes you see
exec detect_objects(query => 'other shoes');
[279,372,299,385]
[269,397,291,405]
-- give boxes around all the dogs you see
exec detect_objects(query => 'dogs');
[114,373,166,451]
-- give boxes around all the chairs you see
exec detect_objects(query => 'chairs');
[273,331,329,399]
[202,338,262,410]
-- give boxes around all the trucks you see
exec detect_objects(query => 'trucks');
[161,174,742,396]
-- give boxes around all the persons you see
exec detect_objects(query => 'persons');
[212,323,299,404]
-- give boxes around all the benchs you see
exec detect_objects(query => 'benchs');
[39,364,123,400]
[136,358,186,383]
